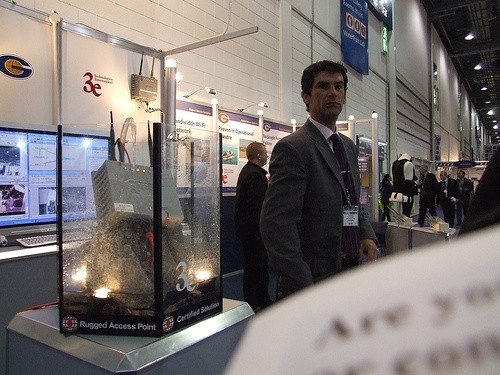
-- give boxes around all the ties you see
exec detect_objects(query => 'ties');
[422,175,425,180]
[329,134,360,259]
[443,182,446,189]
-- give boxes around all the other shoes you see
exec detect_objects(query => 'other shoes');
[251,305,262,313]
[457,223,462,226]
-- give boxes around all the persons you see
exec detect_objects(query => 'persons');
[223,153,500,375]
[234,142,271,311]
[259,60,380,300]
